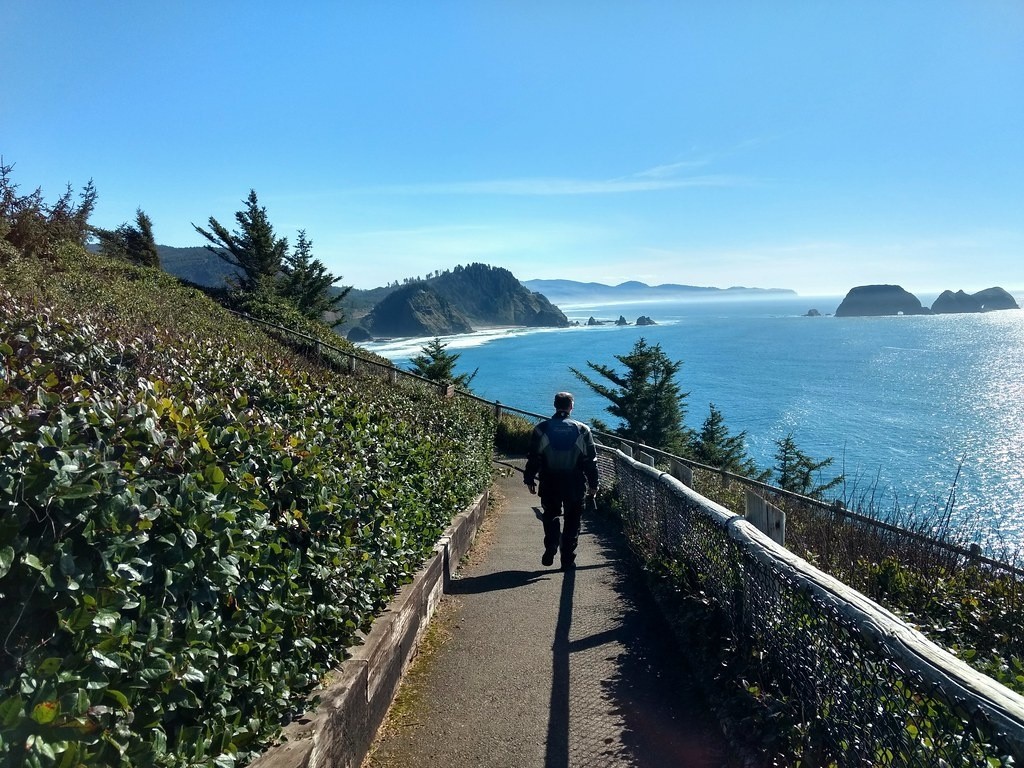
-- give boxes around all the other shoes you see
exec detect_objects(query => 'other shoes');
[542,543,558,566]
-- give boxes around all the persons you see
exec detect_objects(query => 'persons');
[523,392,600,568]
